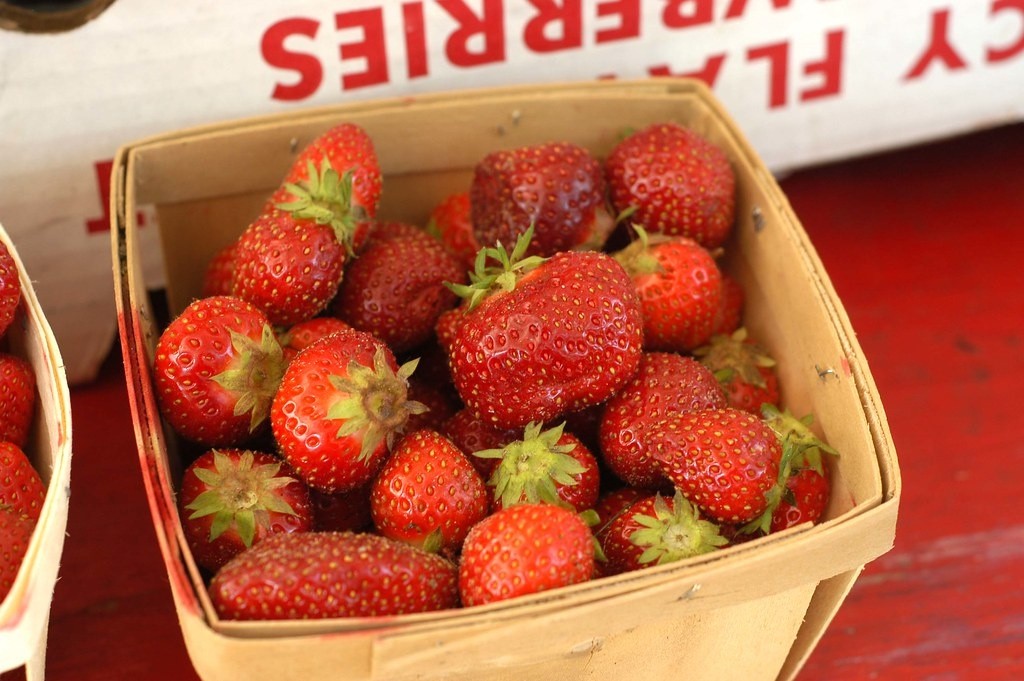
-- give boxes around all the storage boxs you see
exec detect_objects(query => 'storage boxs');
[109,76,903,681]
[0,222,71,681]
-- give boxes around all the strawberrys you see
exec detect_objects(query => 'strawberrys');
[154,121,844,622]
[0,240,47,606]
